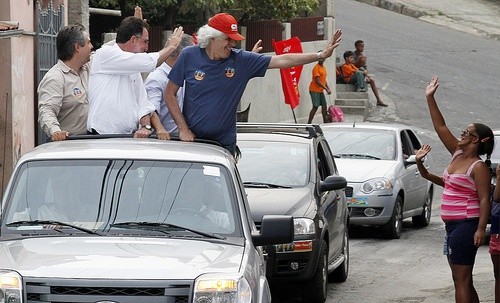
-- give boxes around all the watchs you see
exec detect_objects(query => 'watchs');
[317,52,321,60]
[141,124,152,132]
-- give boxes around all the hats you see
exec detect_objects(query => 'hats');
[208,11,246,40]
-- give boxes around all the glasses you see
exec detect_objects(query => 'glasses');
[461,128,479,137]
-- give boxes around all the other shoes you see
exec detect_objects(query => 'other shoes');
[357,88,366,92]
[377,100,388,106]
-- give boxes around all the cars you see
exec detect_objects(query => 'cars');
[315,123,435,239]
[0,134,295,303]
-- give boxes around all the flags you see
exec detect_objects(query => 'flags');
[271,37,304,109]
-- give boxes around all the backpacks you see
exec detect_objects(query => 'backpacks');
[328,105,343,121]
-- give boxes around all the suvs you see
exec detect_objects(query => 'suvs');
[228,122,352,301]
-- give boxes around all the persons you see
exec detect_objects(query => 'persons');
[37,5,146,141]
[165,12,342,162]
[352,40,388,107]
[145,32,195,140]
[307,50,332,124]
[341,51,369,92]
[158,166,230,229]
[416,75,500,303]
[261,147,324,183]
[86,16,184,139]
[368,136,409,161]
[11,170,103,224]
